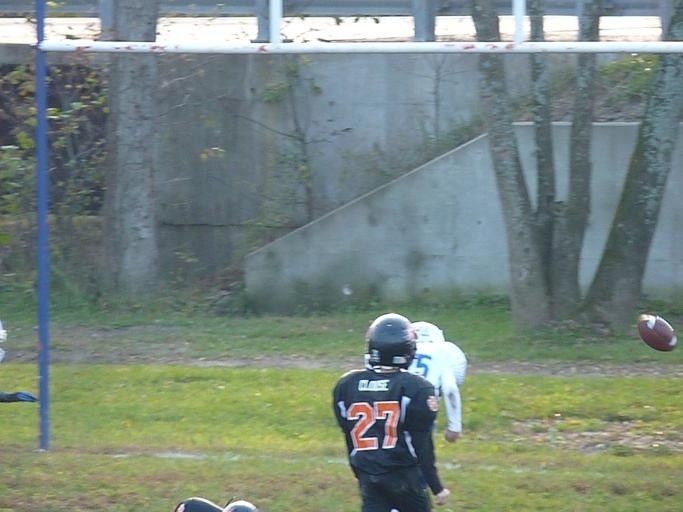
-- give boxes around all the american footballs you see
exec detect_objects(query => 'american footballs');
[639,314,677,351]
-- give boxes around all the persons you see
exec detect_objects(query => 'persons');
[175,497,256,512]
[407,320,467,442]
[333,313,450,512]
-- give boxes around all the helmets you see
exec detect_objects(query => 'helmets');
[412,321,445,343]
[364,313,417,370]
